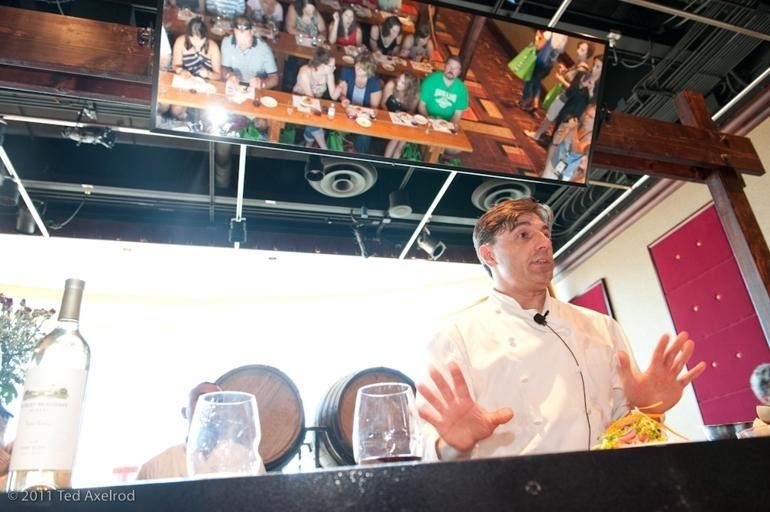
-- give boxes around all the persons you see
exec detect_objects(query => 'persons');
[158,0,469,162]
[134,382,267,482]
[415,199,708,465]
[514,29,602,182]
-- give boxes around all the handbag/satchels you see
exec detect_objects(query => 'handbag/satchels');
[507,42,537,83]
[542,80,562,110]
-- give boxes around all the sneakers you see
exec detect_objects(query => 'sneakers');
[523,127,540,140]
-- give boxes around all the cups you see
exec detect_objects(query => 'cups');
[351,382,423,465]
[186,391,262,478]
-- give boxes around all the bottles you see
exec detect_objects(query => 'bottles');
[6,279,90,493]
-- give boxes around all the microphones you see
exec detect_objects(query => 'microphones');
[534,309,550,328]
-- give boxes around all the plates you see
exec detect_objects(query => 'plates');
[177,5,455,135]
